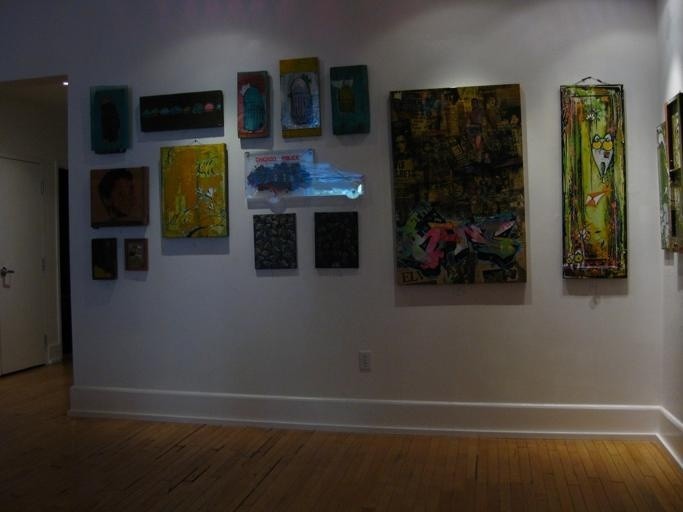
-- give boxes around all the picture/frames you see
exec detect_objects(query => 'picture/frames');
[91,165,151,280]
